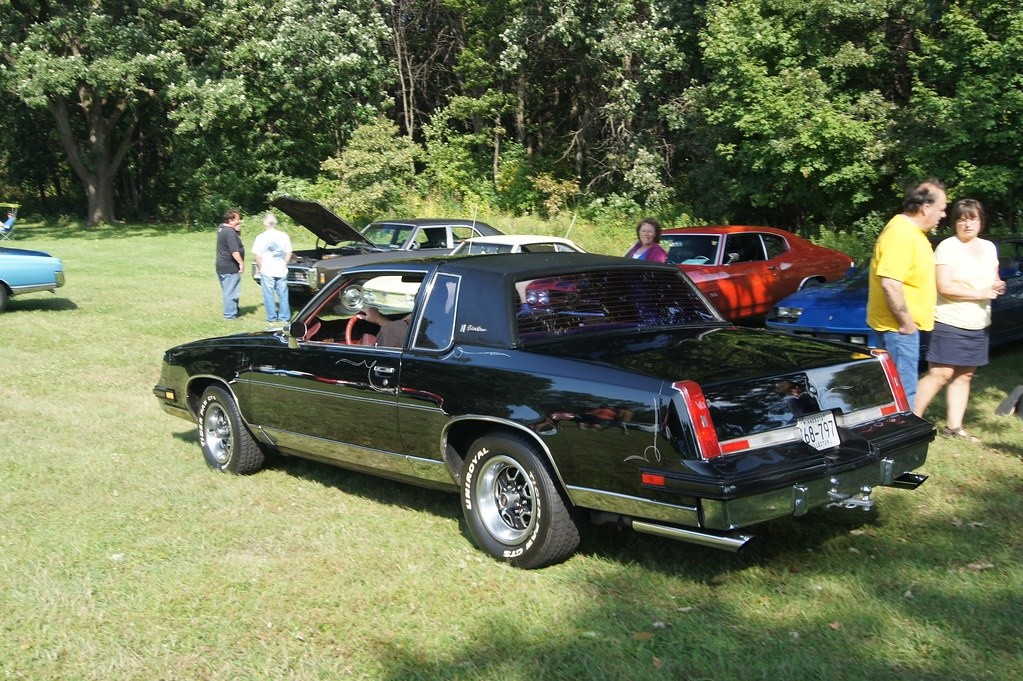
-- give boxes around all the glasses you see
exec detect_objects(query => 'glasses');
[956,218,981,226]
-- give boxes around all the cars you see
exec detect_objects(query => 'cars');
[0,246,66,313]
[623,224,855,321]
[252,197,505,305]
[765,232,1022,360]
[448,234,587,256]
[152,251,937,570]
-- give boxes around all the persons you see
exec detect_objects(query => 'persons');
[252,213,292,323]
[355,279,448,349]
[0,211,16,233]
[865,179,947,414]
[629,217,668,265]
[915,199,1006,445]
[216,210,244,319]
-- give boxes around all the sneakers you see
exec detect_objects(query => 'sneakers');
[941,426,981,444]
